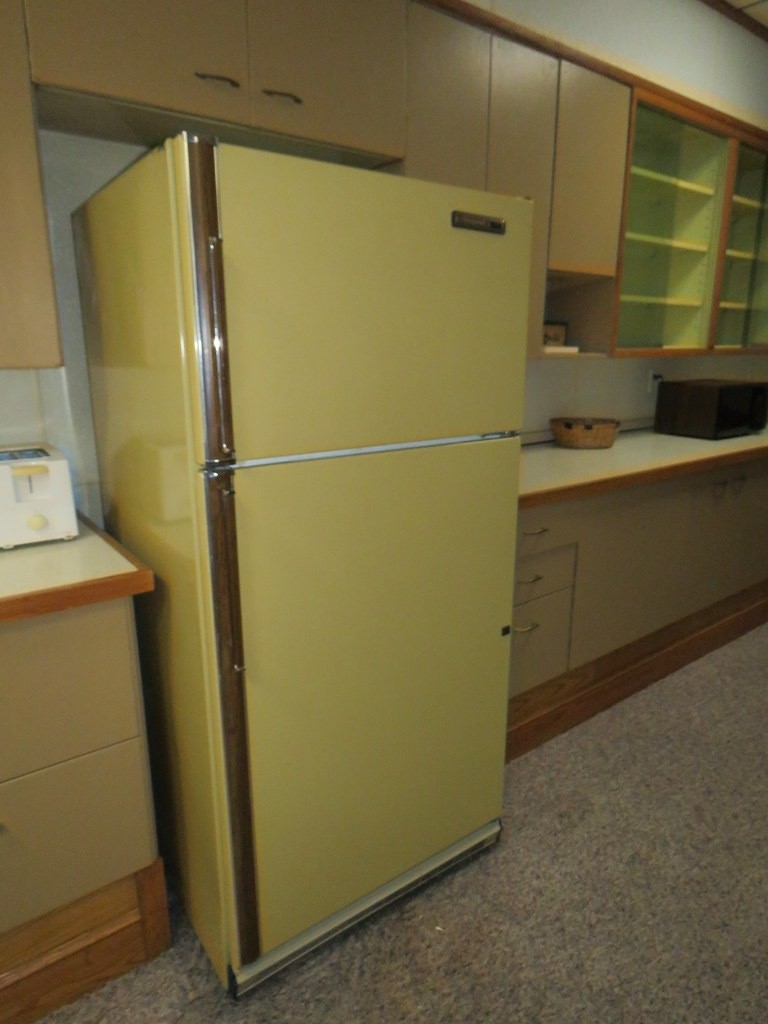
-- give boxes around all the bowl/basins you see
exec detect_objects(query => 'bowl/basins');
[549,418,621,448]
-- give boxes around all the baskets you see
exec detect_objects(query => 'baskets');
[549,417,621,449]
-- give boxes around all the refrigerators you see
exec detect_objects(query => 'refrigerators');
[72,129,534,999]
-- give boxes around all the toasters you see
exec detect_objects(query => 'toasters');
[0,441,80,549]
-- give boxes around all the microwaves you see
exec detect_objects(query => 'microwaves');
[653,374,768,440]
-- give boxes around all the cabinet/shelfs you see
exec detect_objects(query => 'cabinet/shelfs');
[501,493,596,703]
[410,1,636,282]
[548,78,767,360]
[31,0,408,164]
[0,507,172,1024]
